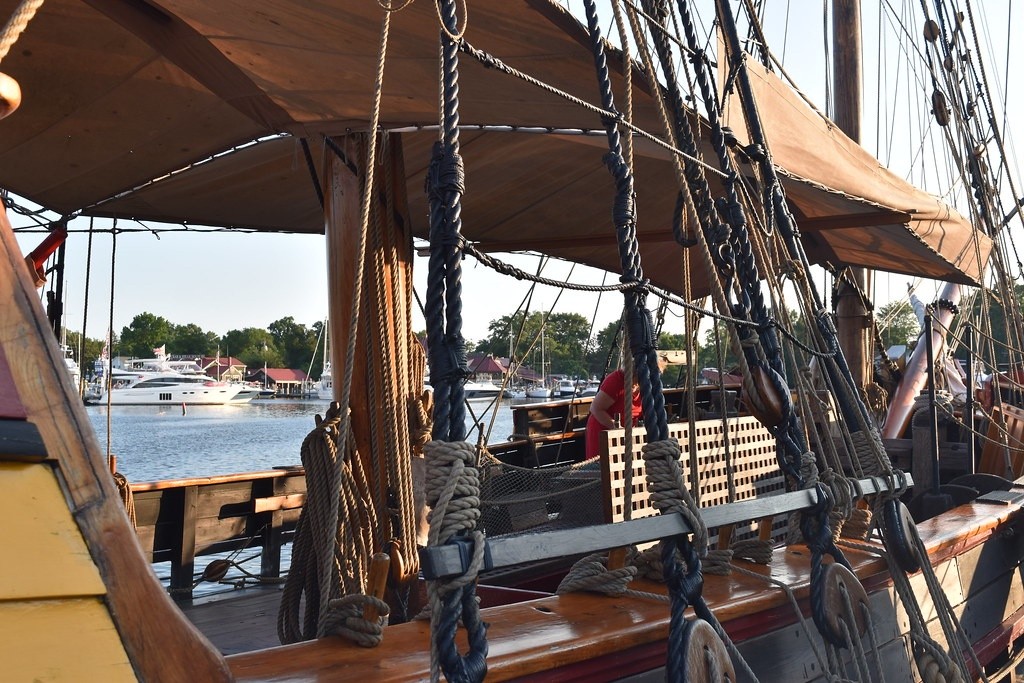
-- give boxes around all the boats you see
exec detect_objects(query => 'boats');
[65,313,603,408]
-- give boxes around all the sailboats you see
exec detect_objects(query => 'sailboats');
[0,1,1024,682]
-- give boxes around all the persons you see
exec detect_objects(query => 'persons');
[552,377,558,386]
[576,353,667,526]
